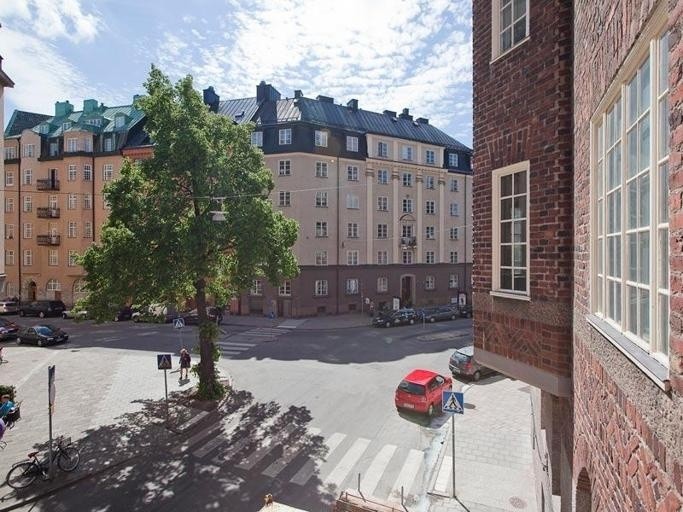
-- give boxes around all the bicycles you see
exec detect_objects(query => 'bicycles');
[6,436,84,489]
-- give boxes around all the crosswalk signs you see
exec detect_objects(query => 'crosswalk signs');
[157,355,173,370]
[440,388,466,417]
[173,316,184,331]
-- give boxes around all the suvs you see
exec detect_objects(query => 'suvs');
[177,305,222,324]
[132,302,175,324]
[448,345,499,379]
[20,300,66,319]
[372,308,417,329]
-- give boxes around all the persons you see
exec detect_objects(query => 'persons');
[179,349,191,380]
[0,394,13,419]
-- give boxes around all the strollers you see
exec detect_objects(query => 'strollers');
[0,400,13,449]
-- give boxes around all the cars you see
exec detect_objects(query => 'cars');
[418,304,459,323]
[0,301,18,317]
[0,318,21,340]
[462,303,472,318]
[114,306,131,321]
[395,370,450,415]
[61,305,94,319]
[17,324,69,348]
